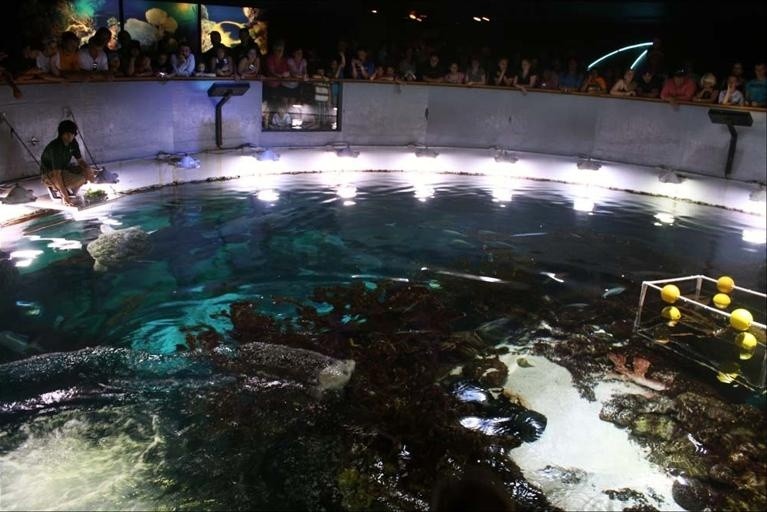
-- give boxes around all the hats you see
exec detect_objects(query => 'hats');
[674,66,688,76]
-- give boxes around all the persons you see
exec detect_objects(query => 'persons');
[1,26,339,129]
[38,119,95,206]
[327,37,767,110]
